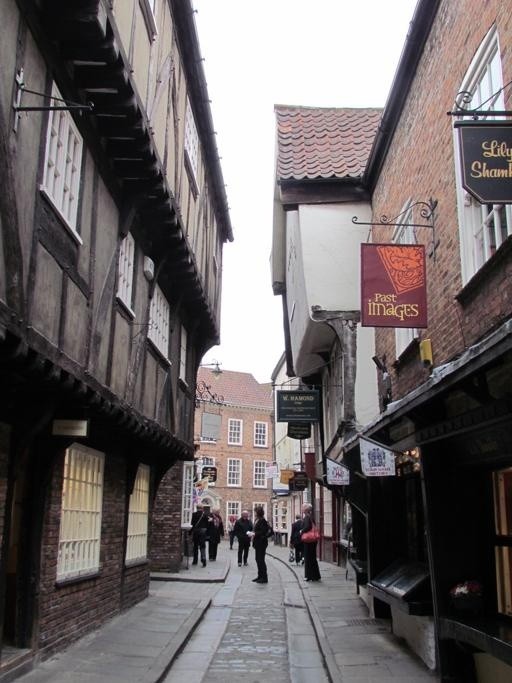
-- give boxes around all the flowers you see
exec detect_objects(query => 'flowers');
[445,579,483,601]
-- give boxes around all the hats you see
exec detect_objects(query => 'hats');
[241,511,248,517]
[197,504,204,509]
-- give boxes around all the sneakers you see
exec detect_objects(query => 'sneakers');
[192,559,206,566]
[238,562,248,566]
[297,557,305,565]
[252,576,268,583]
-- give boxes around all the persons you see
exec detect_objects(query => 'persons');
[247,504,269,583]
[289,513,305,565]
[207,505,225,562]
[190,502,208,568]
[298,502,322,583]
[234,510,254,567]
[227,515,237,549]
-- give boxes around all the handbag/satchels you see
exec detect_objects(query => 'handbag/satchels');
[301,528,320,543]
[266,524,274,538]
[196,528,208,541]
[289,550,295,562]
